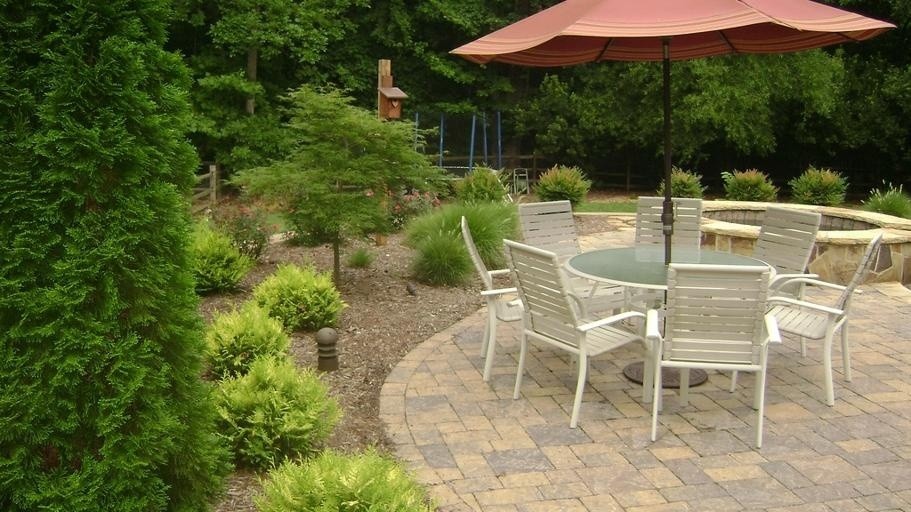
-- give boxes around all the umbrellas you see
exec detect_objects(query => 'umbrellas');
[447,1,899,360]
